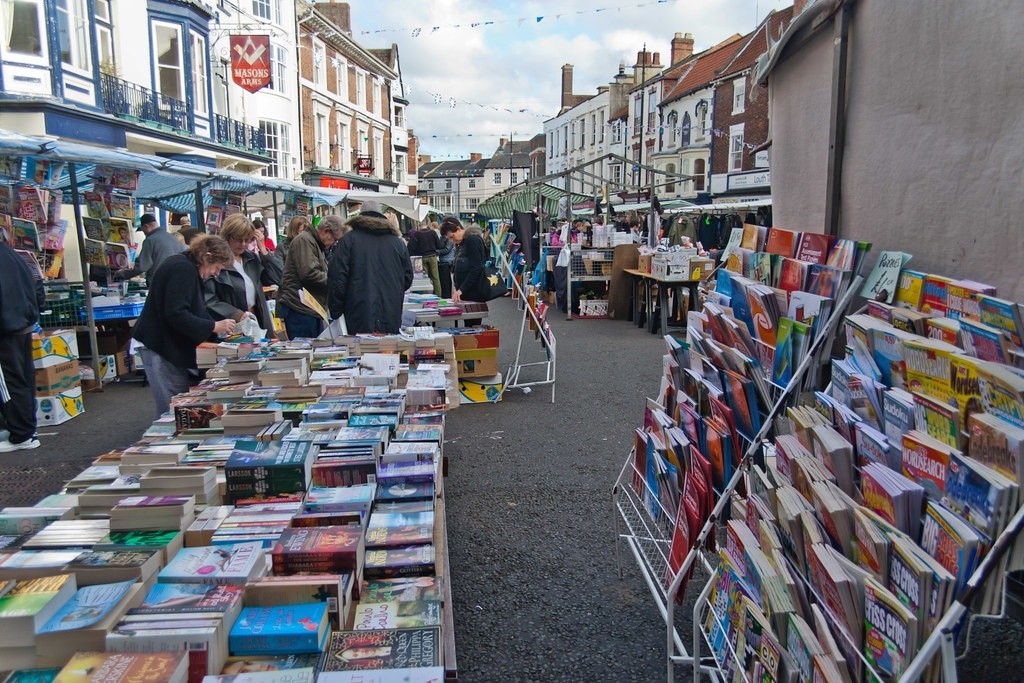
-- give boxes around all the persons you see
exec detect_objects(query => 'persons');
[120,212,488,415]
[219,660,280,675]
[550,219,666,250]
[342,646,393,659]
[0,228,41,453]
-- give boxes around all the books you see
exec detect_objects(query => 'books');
[0,152,149,321]
[631,224,1024,683]
[495,225,553,360]
[0,253,489,683]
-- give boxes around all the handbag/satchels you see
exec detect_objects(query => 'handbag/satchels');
[478,266,508,303]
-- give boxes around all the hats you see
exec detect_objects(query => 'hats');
[135,214,156,233]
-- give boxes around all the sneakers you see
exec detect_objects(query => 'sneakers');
[0,430,41,453]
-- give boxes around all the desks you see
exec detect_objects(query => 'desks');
[624,268,708,338]
[416,311,488,328]
[546,251,613,312]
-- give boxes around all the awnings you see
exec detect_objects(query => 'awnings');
[478,170,778,216]
[0,121,438,222]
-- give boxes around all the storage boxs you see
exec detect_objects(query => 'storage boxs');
[32,329,86,428]
[39,283,148,323]
[580,300,608,316]
[78,327,145,390]
[452,329,503,403]
[549,292,555,304]
[638,248,716,280]
[592,225,633,247]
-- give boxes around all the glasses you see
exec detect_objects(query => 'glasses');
[231,235,252,244]
[330,230,339,244]
